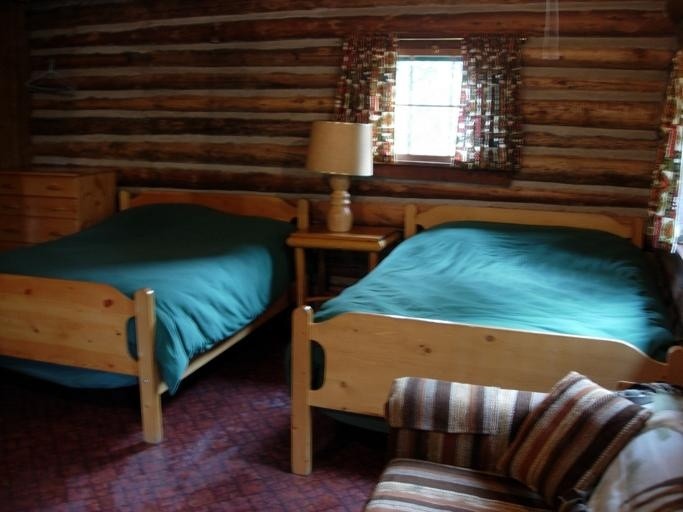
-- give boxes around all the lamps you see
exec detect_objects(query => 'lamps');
[305,119,374,233]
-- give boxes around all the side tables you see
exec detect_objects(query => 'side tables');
[286,224,402,308]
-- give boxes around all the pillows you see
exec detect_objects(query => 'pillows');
[496,369,652,511]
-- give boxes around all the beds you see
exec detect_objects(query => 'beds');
[0,187,309,443]
[288,200,683,475]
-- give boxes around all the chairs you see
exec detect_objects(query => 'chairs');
[361,376,683,511]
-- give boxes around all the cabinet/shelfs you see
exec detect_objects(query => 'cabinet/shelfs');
[0,168,118,250]
